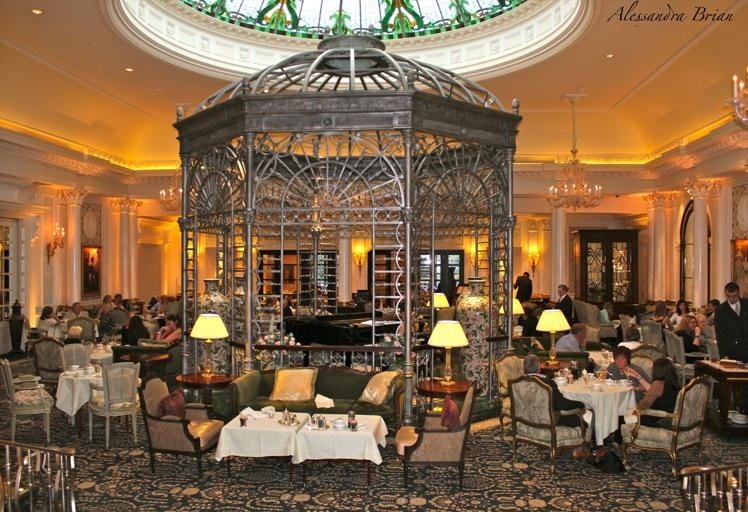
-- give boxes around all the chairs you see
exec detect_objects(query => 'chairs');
[0,290,747,512]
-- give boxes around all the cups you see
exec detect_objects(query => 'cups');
[240,417,247,427]
[349,420,357,432]
[333,418,346,428]
[68,363,101,377]
[95,342,121,351]
[347,410,355,427]
[269,408,274,418]
[552,360,630,391]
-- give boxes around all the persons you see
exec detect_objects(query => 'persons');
[514,272,532,302]
[520,285,719,459]
[37,294,183,349]
[383,304,418,346]
[714,283,748,414]
[267,294,294,316]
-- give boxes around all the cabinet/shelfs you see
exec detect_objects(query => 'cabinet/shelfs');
[365,247,419,309]
[256,247,339,310]
[570,228,644,341]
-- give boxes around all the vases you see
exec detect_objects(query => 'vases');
[189,275,234,377]
[453,275,487,318]
[453,277,499,403]
[224,271,262,377]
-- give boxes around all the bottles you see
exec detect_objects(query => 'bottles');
[314,413,325,429]
[282,407,297,425]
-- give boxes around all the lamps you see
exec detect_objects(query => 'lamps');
[541,91,607,211]
[469,249,482,277]
[723,63,748,128]
[155,162,200,215]
[525,251,541,278]
[352,250,368,279]
[43,219,68,266]
